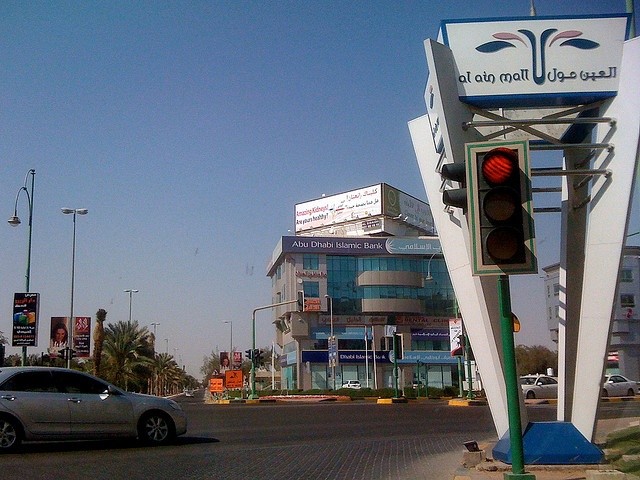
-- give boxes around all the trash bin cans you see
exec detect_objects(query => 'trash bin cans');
[229,389,241,399]
[403,385,418,399]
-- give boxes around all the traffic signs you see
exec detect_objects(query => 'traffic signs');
[212,369,219,375]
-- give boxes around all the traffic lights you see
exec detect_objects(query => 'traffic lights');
[442,162,467,215]
[476,148,527,265]
[298,290,307,312]
[245,350,250,359]
[254,349,264,367]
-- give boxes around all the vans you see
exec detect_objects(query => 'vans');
[413,380,422,389]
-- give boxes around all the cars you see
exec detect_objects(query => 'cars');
[603,374,638,397]
[521,373,558,400]
[0,368,187,454]
[342,380,361,389]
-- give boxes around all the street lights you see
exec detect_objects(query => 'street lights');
[9,170,35,367]
[164,339,168,395]
[152,323,160,343]
[62,209,86,365]
[124,290,138,342]
[225,321,232,370]
[324,295,335,392]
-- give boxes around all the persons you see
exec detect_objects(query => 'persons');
[220,355,230,374]
[51,323,67,355]
